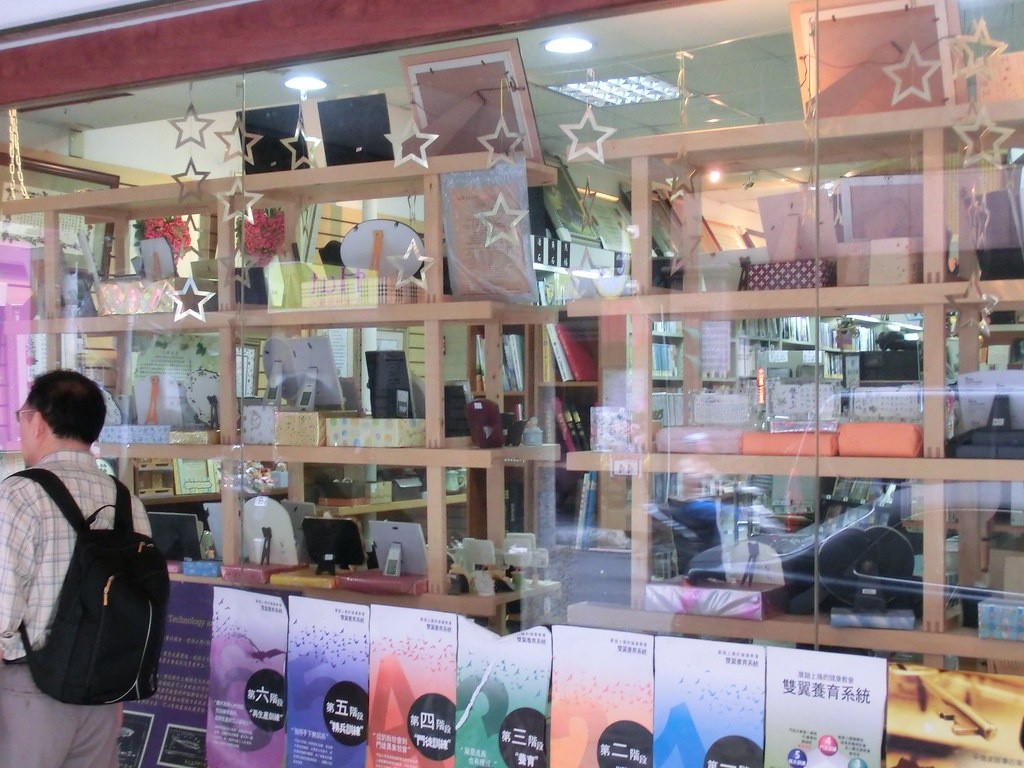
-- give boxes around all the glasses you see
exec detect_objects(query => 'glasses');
[16,409,42,424]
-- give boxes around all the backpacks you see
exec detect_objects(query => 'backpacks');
[1,468,168,706]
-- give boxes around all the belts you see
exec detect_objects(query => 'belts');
[4,656,27,664]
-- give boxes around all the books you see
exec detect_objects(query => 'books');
[652,317,878,375]
[476,322,597,552]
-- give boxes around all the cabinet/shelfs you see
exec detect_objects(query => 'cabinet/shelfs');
[0,100,1024,670]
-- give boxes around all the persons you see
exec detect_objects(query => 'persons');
[0,367,152,768]
